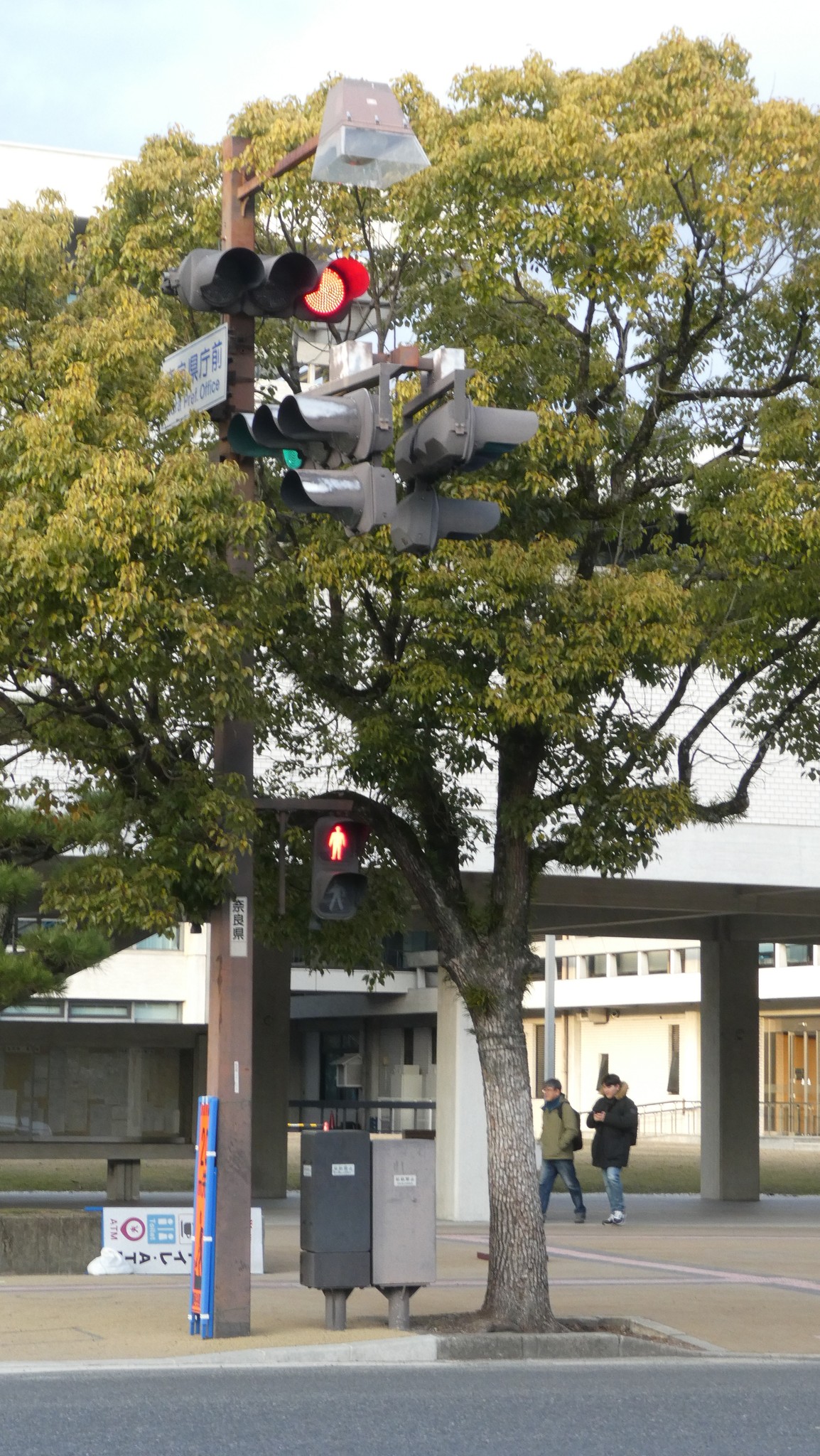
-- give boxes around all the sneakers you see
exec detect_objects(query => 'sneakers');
[602,1214,615,1225]
[575,1214,586,1223]
[613,1210,626,1225]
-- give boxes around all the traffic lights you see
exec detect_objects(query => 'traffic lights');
[225,387,379,470]
[280,461,398,538]
[169,246,370,324]
[413,393,539,477]
[389,483,501,558]
[310,815,371,922]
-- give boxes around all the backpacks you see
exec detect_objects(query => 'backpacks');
[559,1101,582,1151]
[630,1125,638,1146]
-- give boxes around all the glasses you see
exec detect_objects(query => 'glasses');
[542,1088,555,1092]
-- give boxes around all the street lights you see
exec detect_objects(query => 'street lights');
[184,74,432,1339]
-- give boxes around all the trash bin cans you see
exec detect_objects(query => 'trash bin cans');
[298,1127,370,1287]
[106,1158,142,1202]
[370,1137,436,1287]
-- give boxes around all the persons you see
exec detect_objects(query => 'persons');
[586,1074,638,1226]
[537,1078,585,1223]
